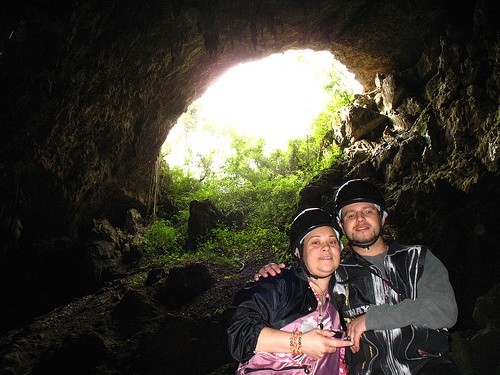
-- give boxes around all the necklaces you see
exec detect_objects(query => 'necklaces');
[307,277,328,296]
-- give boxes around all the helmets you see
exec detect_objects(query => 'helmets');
[334,179,388,217]
[290,208,342,255]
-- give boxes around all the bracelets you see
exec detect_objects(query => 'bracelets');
[290,330,304,355]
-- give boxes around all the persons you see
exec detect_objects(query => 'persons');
[254,179,459,375]
[227,207,354,375]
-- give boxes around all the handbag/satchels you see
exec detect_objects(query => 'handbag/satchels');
[398,325,449,361]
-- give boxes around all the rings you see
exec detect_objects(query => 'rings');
[351,337,354,339]
